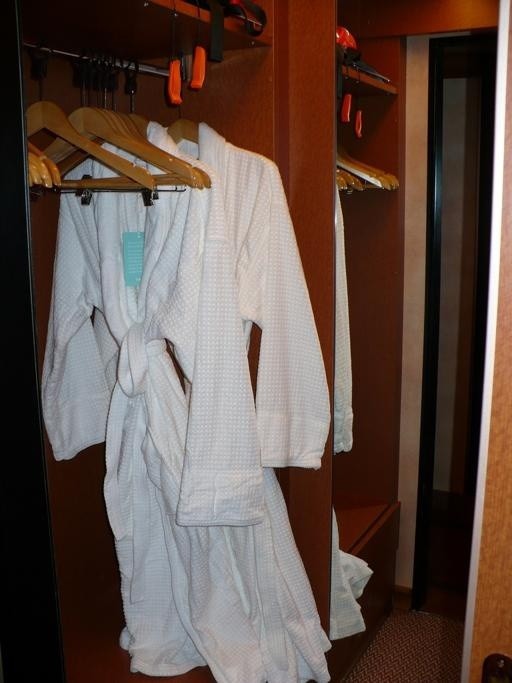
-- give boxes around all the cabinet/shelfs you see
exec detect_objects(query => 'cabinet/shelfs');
[328,500,401,683]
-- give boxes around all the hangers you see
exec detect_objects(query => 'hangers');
[335,140,400,196]
[26,39,216,205]
